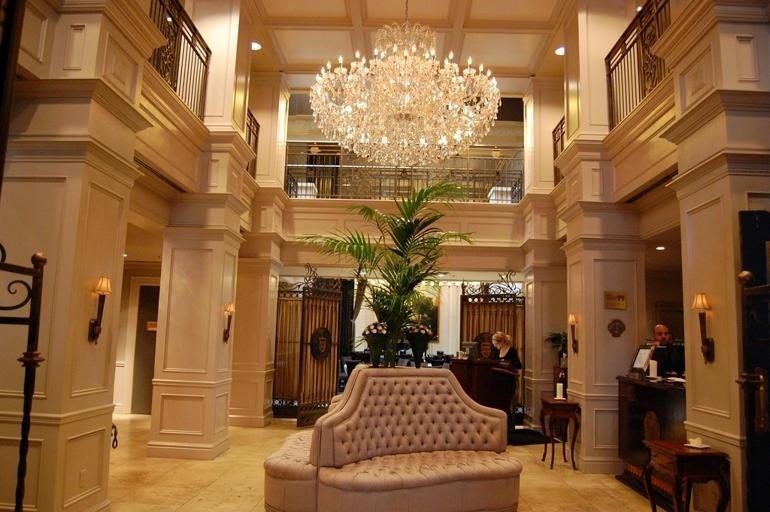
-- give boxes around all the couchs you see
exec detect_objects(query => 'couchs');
[263,361,372,512]
[319,365,522,512]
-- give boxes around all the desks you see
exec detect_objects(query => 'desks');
[538,396,580,469]
[643,437,731,511]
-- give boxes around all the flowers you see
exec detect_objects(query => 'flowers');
[361,322,392,368]
[402,324,434,369]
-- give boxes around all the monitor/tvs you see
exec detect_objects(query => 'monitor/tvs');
[462,342,481,360]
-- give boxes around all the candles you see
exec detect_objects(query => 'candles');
[556,382,564,398]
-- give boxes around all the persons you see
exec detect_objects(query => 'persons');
[477,331,523,370]
[647,324,685,378]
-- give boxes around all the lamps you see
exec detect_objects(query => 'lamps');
[221,303,236,344]
[566,311,579,352]
[689,292,715,363]
[87,276,114,344]
[308,1,501,167]
